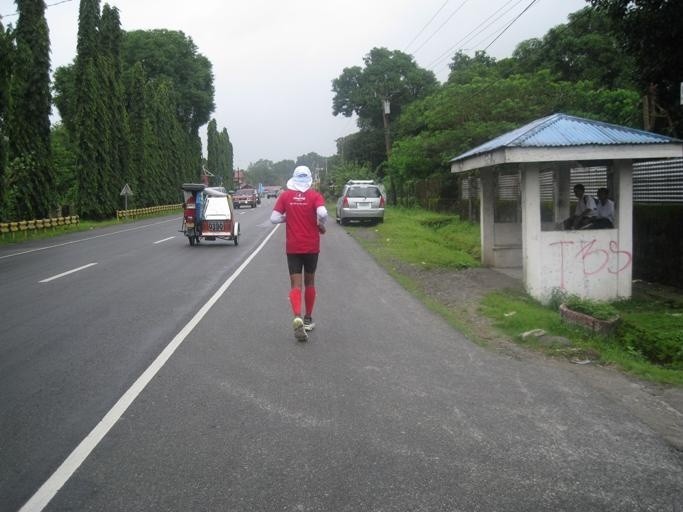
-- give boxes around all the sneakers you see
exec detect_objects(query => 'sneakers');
[292,317,309,341]
[303,316,315,331]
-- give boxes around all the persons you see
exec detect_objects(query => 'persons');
[181,190,196,208]
[269,164,327,342]
[562,181,596,230]
[377,179,385,203]
[580,188,615,231]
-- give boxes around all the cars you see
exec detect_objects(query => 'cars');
[267,190,278,199]
[335,179,385,226]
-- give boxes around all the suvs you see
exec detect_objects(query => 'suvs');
[232,187,260,208]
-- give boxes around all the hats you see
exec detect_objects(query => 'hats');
[293,165,312,181]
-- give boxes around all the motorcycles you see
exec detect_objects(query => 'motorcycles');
[180,182,240,244]
